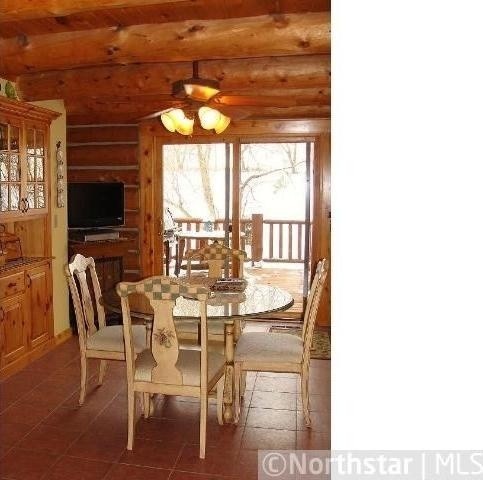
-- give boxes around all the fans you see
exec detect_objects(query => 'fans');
[134,60,296,121]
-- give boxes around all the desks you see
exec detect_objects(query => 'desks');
[98,278,293,423]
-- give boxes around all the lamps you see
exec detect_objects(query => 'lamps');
[160,83,231,136]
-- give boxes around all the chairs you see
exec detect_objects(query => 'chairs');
[63,253,146,406]
[232,257,330,429]
[114,276,227,460]
[184,240,247,278]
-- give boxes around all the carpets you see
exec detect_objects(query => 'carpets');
[268,325,330,359]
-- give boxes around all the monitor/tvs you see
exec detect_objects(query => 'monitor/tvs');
[67,181,125,229]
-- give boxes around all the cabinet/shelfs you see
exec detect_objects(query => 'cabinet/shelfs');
[0,96,63,219]
[67,233,139,337]
[0,263,54,383]
